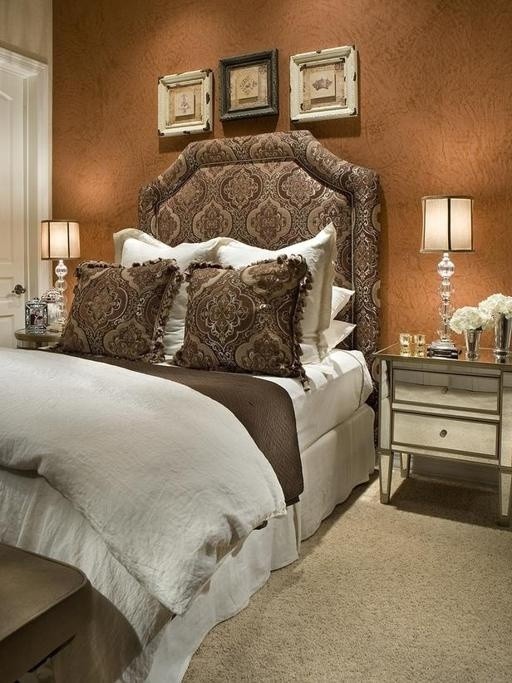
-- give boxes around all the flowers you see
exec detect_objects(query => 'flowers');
[448,305,487,335]
[478,293,512,324]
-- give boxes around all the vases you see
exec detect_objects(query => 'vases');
[463,329,481,358]
[493,315,511,364]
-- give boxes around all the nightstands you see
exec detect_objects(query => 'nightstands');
[15,324,66,353]
[371,340,512,521]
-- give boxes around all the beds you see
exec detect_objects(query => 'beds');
[0,128,381,677]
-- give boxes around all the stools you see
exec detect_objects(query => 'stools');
[2,540,96,683]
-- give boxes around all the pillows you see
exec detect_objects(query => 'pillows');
[317,321,358,356]
[46,259,180,364]
[329,285,356,323]
[209,222,338,364]
[170,255,313,390]
[111,229,219,363]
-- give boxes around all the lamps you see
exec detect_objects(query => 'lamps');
[39,219,81,324]
[418,194,475,358]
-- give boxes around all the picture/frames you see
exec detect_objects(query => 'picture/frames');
[289,44,360,125]
[218,48,279,122]
[24,302,48,334]
[157,69,213,138]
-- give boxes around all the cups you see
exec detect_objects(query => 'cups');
[400,347,411,357]
[415,347,427,358]
[414,334,425,346]
[400,333,412,347]
